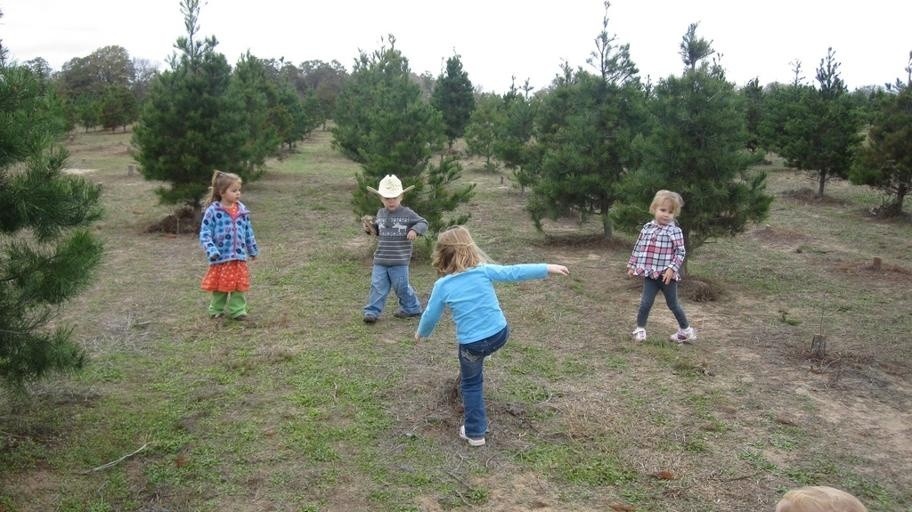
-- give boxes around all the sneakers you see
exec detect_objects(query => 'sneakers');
[235,314,249,321]
[460,425,486,446]
[364,314,376,322]
[395,309,422,318]
[670,328,696,342]
[633,327,646,342]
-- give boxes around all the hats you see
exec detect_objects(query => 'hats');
[367,174,416,198]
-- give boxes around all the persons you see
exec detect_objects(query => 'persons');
[414,223,570,444]
[199,168,260,321]
[775,485,868,512]
[627,188,697,342]
[361,173,430,322]
